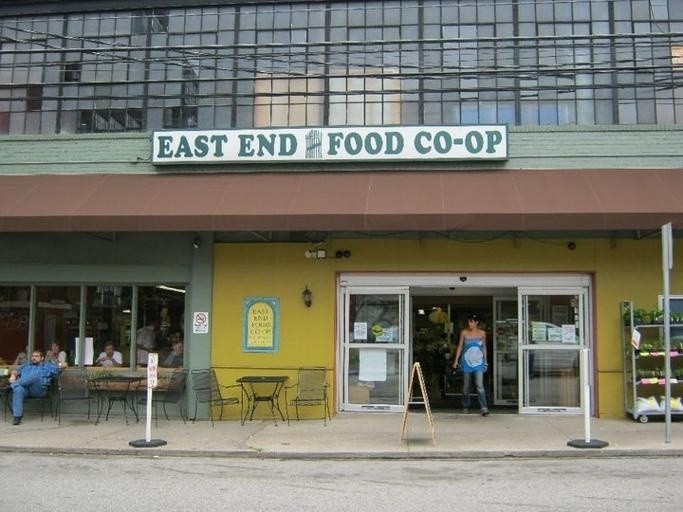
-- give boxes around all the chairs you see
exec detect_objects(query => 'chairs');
[105,378,140,426]
[190,369,239,428]
[138,368,189,428]
[4,375,59,423]
[283,367,332,427]
[86,380,106,427]
[55,368,94,427]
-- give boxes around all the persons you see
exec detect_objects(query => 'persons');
[156,341,183,368]
[453,313,490,415]
[91,340,124,367]
[7,348,68,426]
[14,344,28,365]
[415,326,457,401]
[43,338,68,366]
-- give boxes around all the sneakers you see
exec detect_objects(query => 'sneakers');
[463,408,469,413]
[481,407,489,415]
[0,384,12,393]
[12,413,23,425]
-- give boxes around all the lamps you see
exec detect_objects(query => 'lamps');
[304,249,316,258]
[302,286,312,307]
[193,238,202,249]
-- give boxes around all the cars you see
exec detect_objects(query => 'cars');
[527,321,580,376]
[345,360,404,398]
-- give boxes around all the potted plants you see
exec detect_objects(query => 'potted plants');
[625,309,682,383]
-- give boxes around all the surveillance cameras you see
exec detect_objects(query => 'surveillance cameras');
[304,249,313,258]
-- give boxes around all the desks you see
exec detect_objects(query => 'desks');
[234,376,288,427]
[93,376,146,399]
